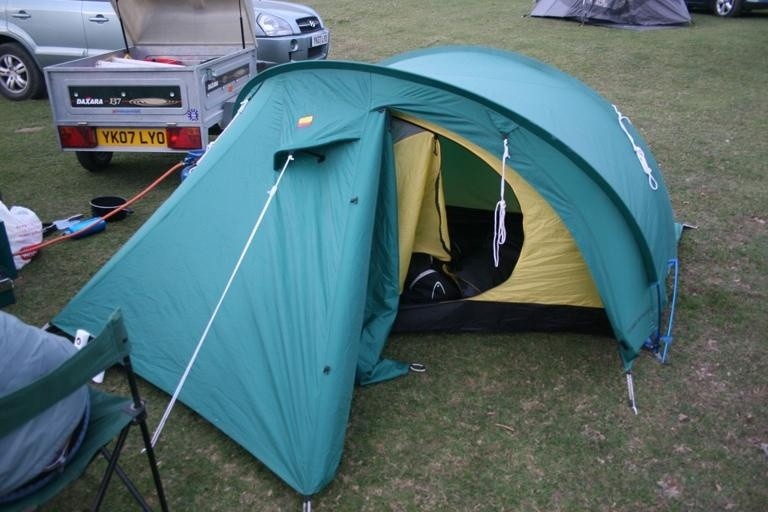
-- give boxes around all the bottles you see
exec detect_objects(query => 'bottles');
[62,216,106,240]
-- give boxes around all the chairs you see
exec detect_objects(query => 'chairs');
[0,311,169,512]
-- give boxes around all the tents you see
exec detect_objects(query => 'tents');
[48,39,698,512]
[522,0,697,28]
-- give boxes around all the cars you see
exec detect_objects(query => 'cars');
[0,0,330,102]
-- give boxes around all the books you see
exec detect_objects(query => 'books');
[71,329,104,384]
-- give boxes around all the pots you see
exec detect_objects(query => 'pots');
[89,195,134,222]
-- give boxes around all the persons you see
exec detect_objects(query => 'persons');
[0,311,90,503]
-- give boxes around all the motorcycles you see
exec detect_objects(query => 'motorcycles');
[42,0,258,172]
